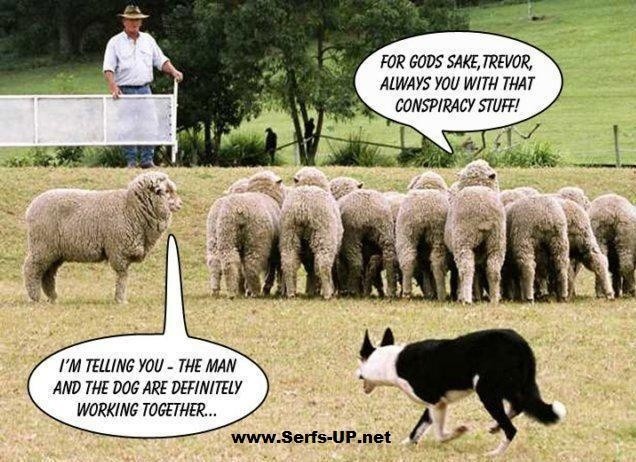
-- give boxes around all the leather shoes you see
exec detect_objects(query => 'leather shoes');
[141,162,155,168]
[128,162,137,168]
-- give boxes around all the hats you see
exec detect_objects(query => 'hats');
[116,5,149,19]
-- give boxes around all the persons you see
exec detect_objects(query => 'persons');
[102,5,183,168]
[266,128,277,164]
[304,118,314,154]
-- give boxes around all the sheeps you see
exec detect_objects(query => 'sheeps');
[23,170,184,304]
[206,158,635,306]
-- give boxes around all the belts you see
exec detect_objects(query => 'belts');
[118,83,146,88]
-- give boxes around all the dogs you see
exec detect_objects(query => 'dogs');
[357,326,566,457]
